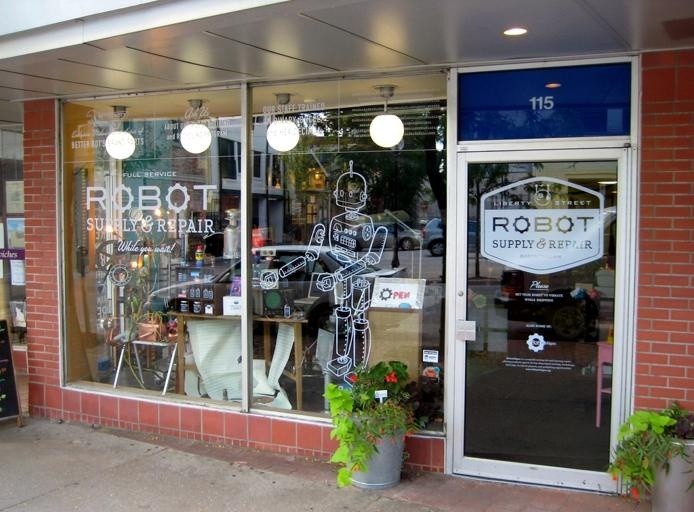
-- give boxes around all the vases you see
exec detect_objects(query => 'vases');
[138,319,164,342]
[352,413,404,489]
[652,414,694,512]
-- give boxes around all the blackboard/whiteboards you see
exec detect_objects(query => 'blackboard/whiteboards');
[1,320,22,418]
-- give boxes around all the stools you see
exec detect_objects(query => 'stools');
[113,339,178,395]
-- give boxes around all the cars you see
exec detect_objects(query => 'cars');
[345,209,421,250]
[140,244,407,316]
[492,270,600,344]
[424,219,482,258]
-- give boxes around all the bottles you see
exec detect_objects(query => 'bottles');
[179,299,189,313]
[223,212,240,260]
[194,245,205,268]
[193,302,202,314]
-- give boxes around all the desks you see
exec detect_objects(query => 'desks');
[166,309,310,410]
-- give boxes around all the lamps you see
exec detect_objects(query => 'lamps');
[368,86,404,148]
[267,93,300,152]
[180,98,212,155]
[105,106,136,158]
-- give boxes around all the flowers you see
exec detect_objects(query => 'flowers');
[324,362,419,486]
[608,410,668,498]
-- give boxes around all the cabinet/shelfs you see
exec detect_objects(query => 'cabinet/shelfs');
[595,341,613,427]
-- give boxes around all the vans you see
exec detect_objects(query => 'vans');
[561,208,613,316]
